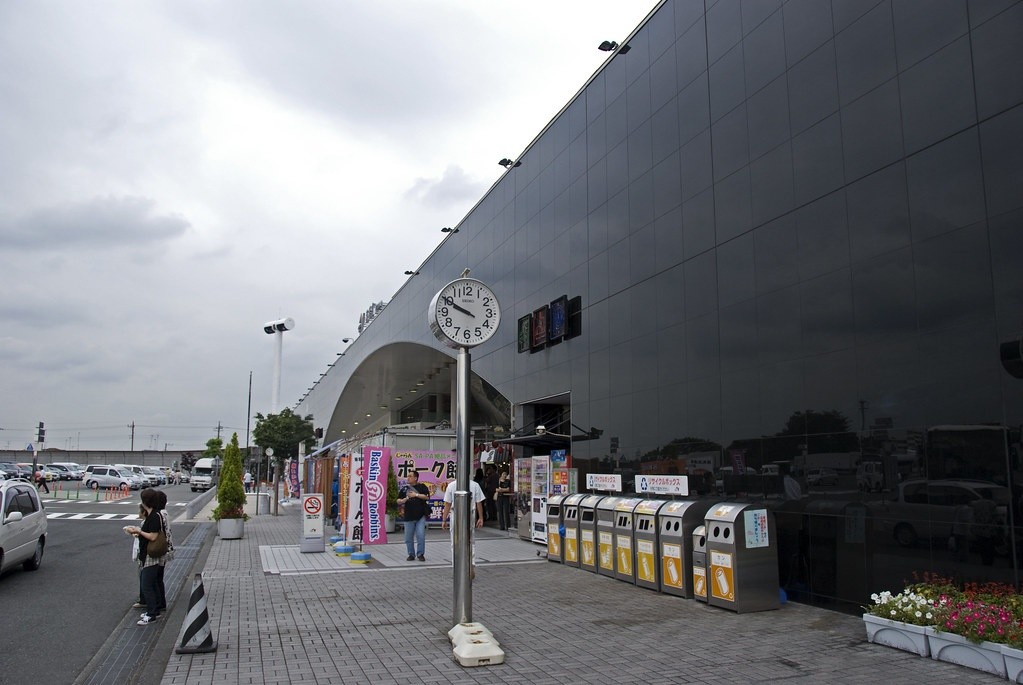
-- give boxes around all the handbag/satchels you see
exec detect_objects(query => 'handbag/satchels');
[493,478,500,501]
[147,532,168,558]
[423,503,432,516]
[132,537,139,562]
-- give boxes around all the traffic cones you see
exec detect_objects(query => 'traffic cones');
[174,572,219,655]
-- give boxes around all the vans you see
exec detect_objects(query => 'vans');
[190,457,220,492]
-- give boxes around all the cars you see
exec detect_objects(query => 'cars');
[0,460,190,490]
[708,459,1023,561]
[0,477,48,578]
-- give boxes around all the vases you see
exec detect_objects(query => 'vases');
[1001,644,1023,685]
[862,612,931,658]
[927,625,1010,679]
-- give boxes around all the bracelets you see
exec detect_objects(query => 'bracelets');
[442,520,446,522]
[138,528,141,533]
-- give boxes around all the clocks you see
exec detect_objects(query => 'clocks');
[427,277,502,349]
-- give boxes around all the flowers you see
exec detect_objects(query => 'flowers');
[858,571,1023,650]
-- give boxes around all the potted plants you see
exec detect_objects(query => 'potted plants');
[212,433,251,539]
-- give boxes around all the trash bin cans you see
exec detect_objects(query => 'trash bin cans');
[563,493,588,567]
[658,500,704,599]
[546,494,572,562]
[703,502,780,613]
[578,494,606,573]
[633,500,668,592]
[692,525,708,602]
[614,498,645,584]
[597,496,624,578]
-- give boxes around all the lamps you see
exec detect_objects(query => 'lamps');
[343,338,354,343]
[599,41,616,51]
[409,362,449,392]
[498,158,513,166]
[405,271,413,274]
[441,228,452,232]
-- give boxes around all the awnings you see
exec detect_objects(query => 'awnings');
[493,427,570,446]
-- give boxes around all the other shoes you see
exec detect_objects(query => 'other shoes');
[137,616,157,626]
[407,556,414,561]
[249,491,251,492]
[501,527,505,529]
[246,491,248,493]
[44,492,50,494]
[133,602,147,607]
[141,611,161,619]
[418,554,425,561]
[507,527,511,530]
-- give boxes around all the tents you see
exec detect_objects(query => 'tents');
[849,443,886,470]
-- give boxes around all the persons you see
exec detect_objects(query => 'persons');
[494,469,511,530]
[174,470,182,486]
[951,487,1004,567]
[121,488,175,625]
[253,473,260,493]
[163,468,171,486]
[442,468,486,580]
[243,470,252,493]
[484,463,499,521]
[397,471,430,562]
[36,466,49,494]
[474,468,487,522]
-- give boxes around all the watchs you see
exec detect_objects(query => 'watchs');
[415,493,419,497]
